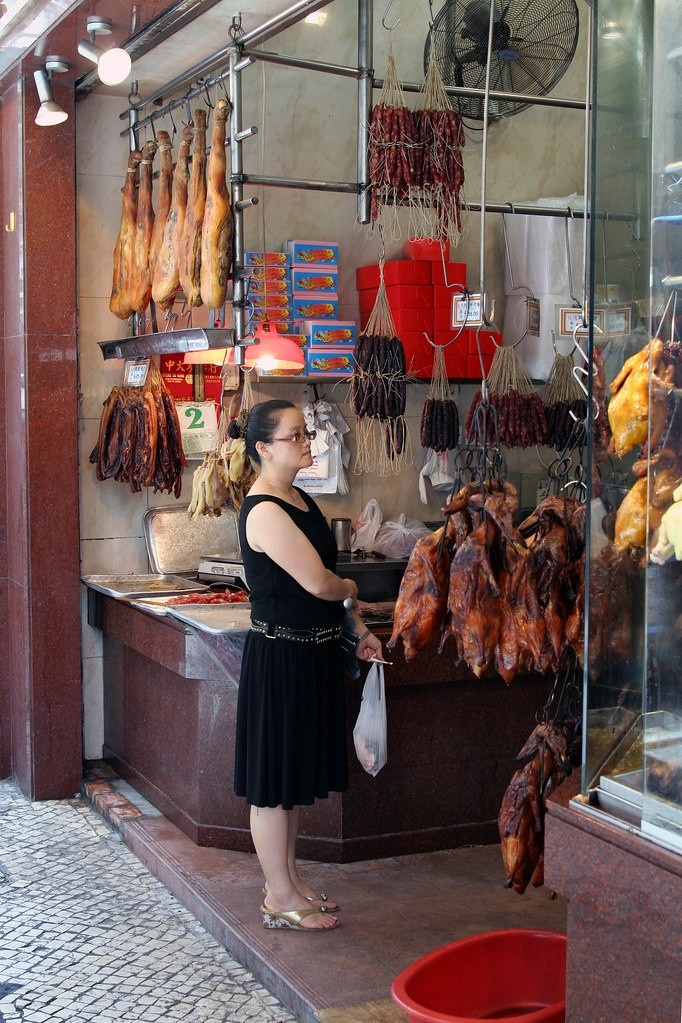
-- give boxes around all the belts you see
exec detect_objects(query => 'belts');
[250,618,361,653]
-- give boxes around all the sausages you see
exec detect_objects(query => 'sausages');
[369,102,465,234]
[349,333,589,462]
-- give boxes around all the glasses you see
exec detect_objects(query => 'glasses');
[264,430,317,443]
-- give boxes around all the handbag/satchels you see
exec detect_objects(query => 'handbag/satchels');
[353,661,387,777]
[350,498,383,553]
[372,513,434,559]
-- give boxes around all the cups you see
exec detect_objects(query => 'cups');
[331,518,356,561]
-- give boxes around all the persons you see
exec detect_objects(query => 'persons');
[226,400,384,931]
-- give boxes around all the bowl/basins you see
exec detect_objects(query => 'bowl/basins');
[390,927,566,1023]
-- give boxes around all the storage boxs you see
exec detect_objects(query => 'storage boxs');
[242,237,504,377]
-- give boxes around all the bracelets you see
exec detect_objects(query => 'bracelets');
[359,629,370,642]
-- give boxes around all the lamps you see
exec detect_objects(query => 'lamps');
[33,54,69,126]
[75,14,130,87]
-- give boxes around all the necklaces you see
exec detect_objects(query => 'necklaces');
[260,476,299,505]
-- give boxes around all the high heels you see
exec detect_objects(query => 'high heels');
[261,909,341,931]
[262,887,340,912]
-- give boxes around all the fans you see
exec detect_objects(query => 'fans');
[424,0,580,121]
